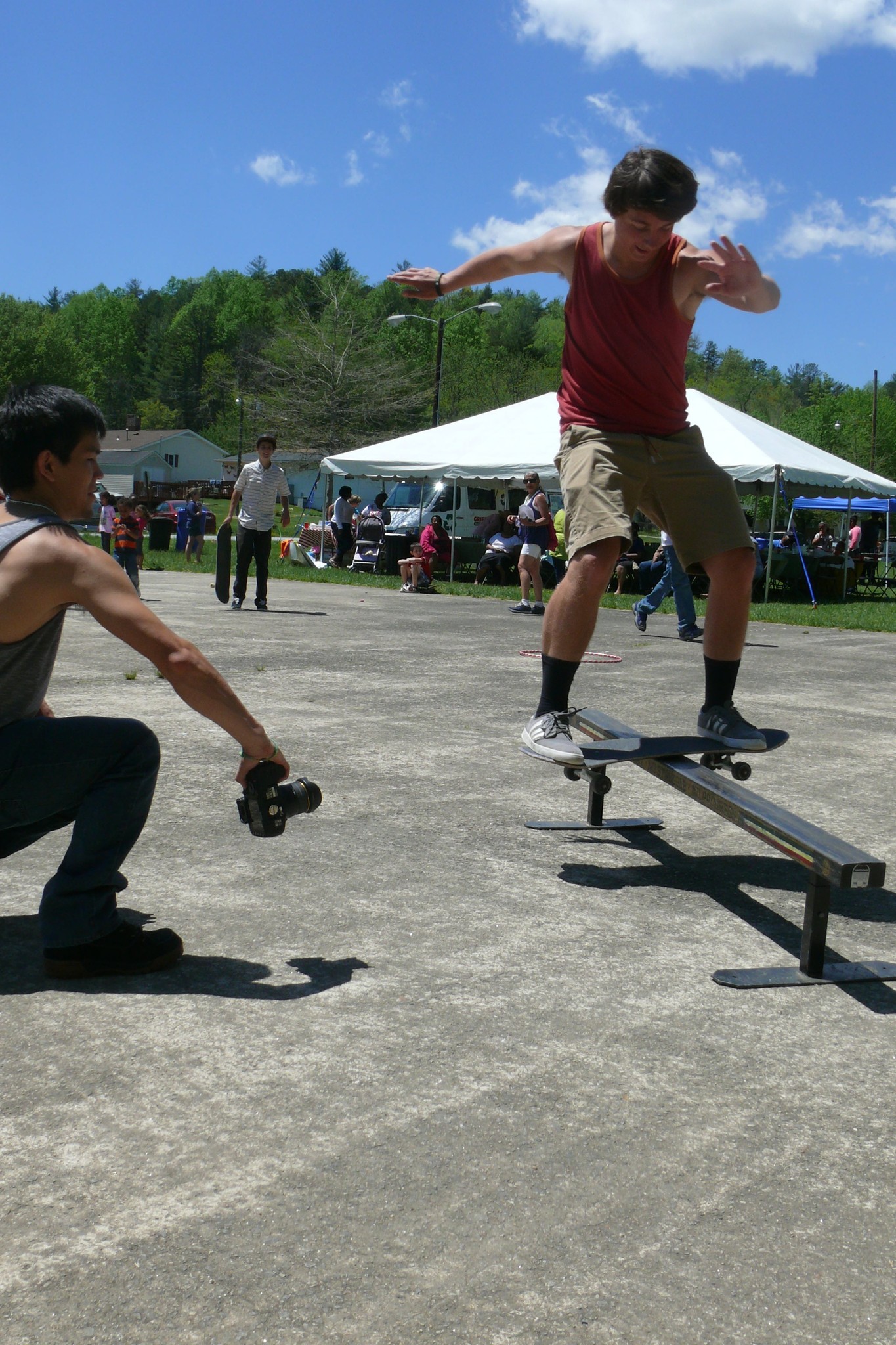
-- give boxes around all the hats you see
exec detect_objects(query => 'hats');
[784,531,794,537]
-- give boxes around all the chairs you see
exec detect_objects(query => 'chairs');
[434,540,708,598]
[759,541,896,598]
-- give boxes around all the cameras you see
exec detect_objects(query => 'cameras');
[236,761,322,838]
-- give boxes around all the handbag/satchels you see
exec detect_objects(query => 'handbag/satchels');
[546,512,558,552]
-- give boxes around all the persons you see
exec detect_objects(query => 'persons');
[383,147,783,766]
[110,497,142,597]
[221,433,291,612]
[844,515,862,595]
[471,469,569,617]
[811,521,833,553]
[328,485,461,593]
[605,521,709,641]
[134,505,148,570]
[183,487,205,565]
[767,530,794,550]
[0,385,293,976]
[98,490,116,556]
[858,511,886,586]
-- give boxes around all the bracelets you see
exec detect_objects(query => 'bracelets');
[238,736,278,761]
[435,272,445,296]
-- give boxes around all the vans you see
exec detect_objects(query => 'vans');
[381,482,565,542]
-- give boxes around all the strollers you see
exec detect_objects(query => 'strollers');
[349,514,386,575]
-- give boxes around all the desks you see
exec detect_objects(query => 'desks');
[298,528,357,561]
[383,532,419,574]
[759,546,813,588]
[853,558,879,596]
[450,541,487,575]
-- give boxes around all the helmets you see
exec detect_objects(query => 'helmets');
[256,434,276,446]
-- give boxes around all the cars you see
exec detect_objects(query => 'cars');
[150,500,216,532]
[90,480,124,503]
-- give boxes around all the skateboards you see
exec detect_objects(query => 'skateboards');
[518,726,791,793]
[210,522,232,604]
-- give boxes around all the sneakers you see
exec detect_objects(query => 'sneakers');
[697,700,766,749]
[632,601,648,632]
[399,583,408,592]
[231,597,243,610]
[524,706,589,765]
[403,583,419,593]
[42,921,184,980]
[508,602,532,614]
[678,625,704,641]
[257,599,268,611]
[530,605,545,616]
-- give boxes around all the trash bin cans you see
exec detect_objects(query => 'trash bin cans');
[148,516,173,552]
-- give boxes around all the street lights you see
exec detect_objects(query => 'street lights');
[387,302,503,429]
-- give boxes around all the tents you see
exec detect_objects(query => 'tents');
[317,388,896,602]
[784,495,896,590]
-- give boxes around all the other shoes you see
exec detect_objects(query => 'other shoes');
[847,589,855,594]
[329,558,342,569]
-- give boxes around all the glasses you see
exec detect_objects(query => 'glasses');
[523,478,538,484]
